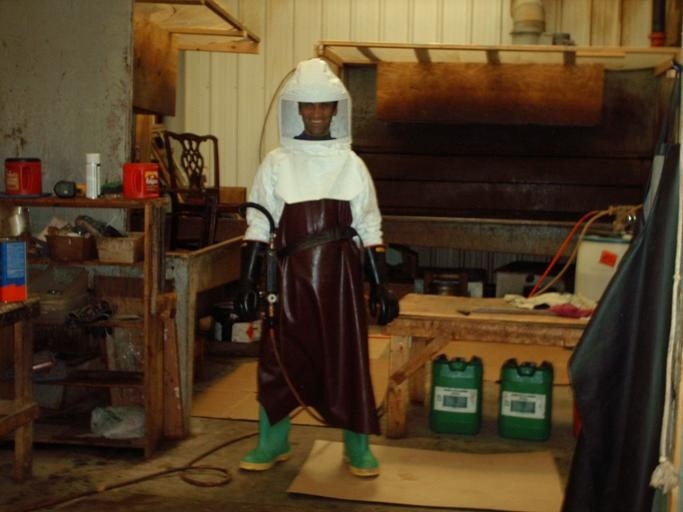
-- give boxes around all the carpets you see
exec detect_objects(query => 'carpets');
[1,412,574,512]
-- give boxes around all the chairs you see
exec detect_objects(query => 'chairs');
[165,130,241,249]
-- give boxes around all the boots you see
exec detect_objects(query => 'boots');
[342,428,379,479]
[239,404,291,471]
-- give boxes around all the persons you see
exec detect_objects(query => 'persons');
[234,58,399,477]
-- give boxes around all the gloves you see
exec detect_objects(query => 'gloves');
[235,246,263,317]
[364,244,400,326]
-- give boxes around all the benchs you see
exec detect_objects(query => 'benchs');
[387,292,600,443]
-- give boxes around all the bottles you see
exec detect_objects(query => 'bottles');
[85,153,101,200]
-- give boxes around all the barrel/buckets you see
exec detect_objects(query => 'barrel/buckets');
[497,357,554,442]
[121,162,160,199]
[3,157,43,197]
[428,354,484,435]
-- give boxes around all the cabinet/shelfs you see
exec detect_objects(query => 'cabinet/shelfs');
[1,198,164,461]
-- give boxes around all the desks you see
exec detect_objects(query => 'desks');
[25,232,243,438]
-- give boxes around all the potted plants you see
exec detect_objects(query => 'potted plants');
[0,120,44,196]
